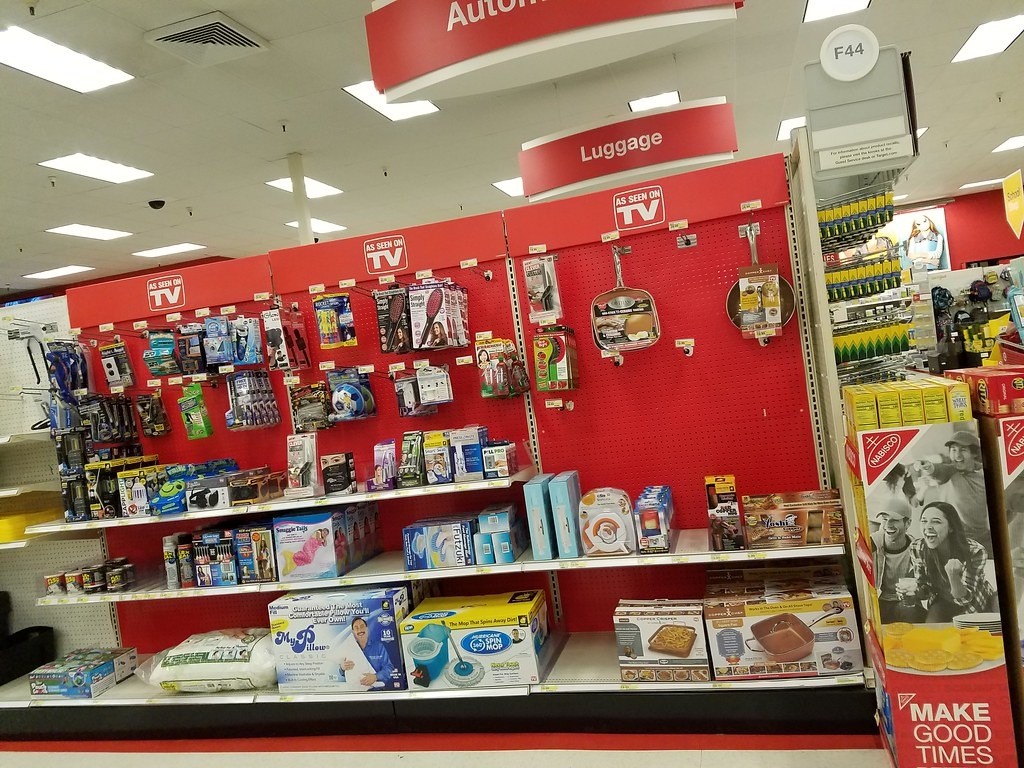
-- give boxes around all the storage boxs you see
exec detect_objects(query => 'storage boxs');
[274,498,383,583]
[402,501,528,572]
[613,598,713,682]
[702,564,866,681]
[56,435,287,522]
[704,474,845,550]
[267,578,440,696]
[635,485,675,555]
[28,645,139,699]
[944,364,1024,417]
[842,374,971,652]
[523,473,557,561]
[321,452,358,496]
[548,471,582,558]
[400,589,552,691]
[422,423,518,484]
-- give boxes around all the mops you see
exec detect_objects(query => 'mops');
[441,619,486,687]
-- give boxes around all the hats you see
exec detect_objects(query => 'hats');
[944,431,980,447]
[875,498,913,520]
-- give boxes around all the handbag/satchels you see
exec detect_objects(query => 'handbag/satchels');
[935,311,953,326]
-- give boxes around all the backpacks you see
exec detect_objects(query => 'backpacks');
[953,309,971,323]
[1002,285,1011,299]
[1001,269,1011,280]
[990,283,1004,300]
[985,270,999,283]
[931,286,955,315]
[969,279,992,304]
[970,307,989,323]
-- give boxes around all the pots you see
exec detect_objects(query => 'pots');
[591,247,661,352]
[726,226,795,343]
[745,607,842,660]
[648,620,695,656]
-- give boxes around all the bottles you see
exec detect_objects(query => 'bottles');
[163,526,217,590]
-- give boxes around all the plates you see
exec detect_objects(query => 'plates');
[625,670,708,680]
[885,654,1005,675]
[953,612,1002,633]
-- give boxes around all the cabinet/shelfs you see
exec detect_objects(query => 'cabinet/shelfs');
[907,255,1024,371]
[0,124,883,740]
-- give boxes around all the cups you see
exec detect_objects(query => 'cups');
[899,579,917,607]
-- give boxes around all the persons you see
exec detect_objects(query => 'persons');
[905,214,944,271]
[913,431,995,561]
[869,500,923,624]
[909,502,995,624]
